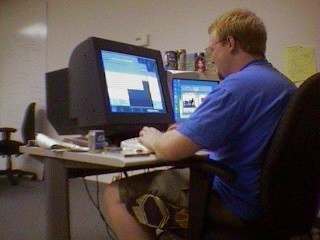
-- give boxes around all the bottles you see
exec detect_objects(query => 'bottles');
[177,49,186,70]
[194,52,205,71]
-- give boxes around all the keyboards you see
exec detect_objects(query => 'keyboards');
[120,137,155,156]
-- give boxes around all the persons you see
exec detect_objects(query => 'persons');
[194,56,205,71]
[101,7,298,240]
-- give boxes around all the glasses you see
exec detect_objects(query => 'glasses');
[205,36,228,57]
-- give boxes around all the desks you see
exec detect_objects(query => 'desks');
[19,144,209,240]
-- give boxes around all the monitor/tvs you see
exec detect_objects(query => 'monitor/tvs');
[46,36,175,147]
[166,68,222,123]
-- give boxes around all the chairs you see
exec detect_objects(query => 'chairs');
[0,103,38,186]
[162,71,320,240]
